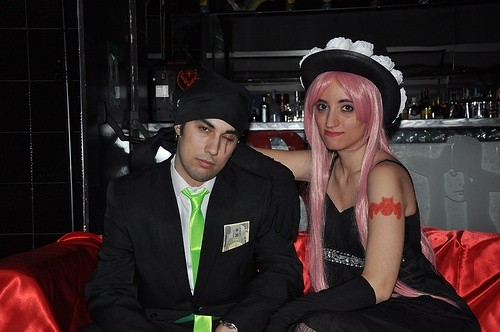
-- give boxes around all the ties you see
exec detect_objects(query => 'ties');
[181,188,213,332]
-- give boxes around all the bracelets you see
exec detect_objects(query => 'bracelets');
[219,320,237,330]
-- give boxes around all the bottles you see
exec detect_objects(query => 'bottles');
[421,93,495,119]
[281,105,289,122]
[259,96,269,123]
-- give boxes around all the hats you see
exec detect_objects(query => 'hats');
[299,37,407,126]
[173,79,252,132]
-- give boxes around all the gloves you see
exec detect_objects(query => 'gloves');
[266,277,376,332]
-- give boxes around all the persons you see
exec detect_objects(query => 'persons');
[83,81,303,332]
[156,38,482,332]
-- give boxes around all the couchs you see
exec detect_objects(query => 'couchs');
[0,227,500,332]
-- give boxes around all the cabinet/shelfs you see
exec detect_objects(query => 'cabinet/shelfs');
[122,0,500,159]
